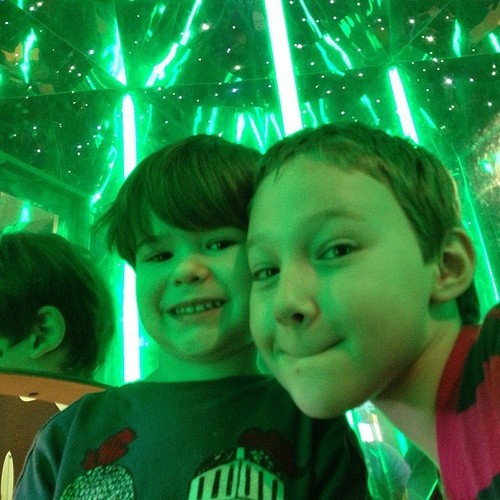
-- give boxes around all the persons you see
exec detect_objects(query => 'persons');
[2,229,122,388]
[244,116,500,499]
[8,132,372,499]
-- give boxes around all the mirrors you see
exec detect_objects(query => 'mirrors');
[0,0,500,500]
